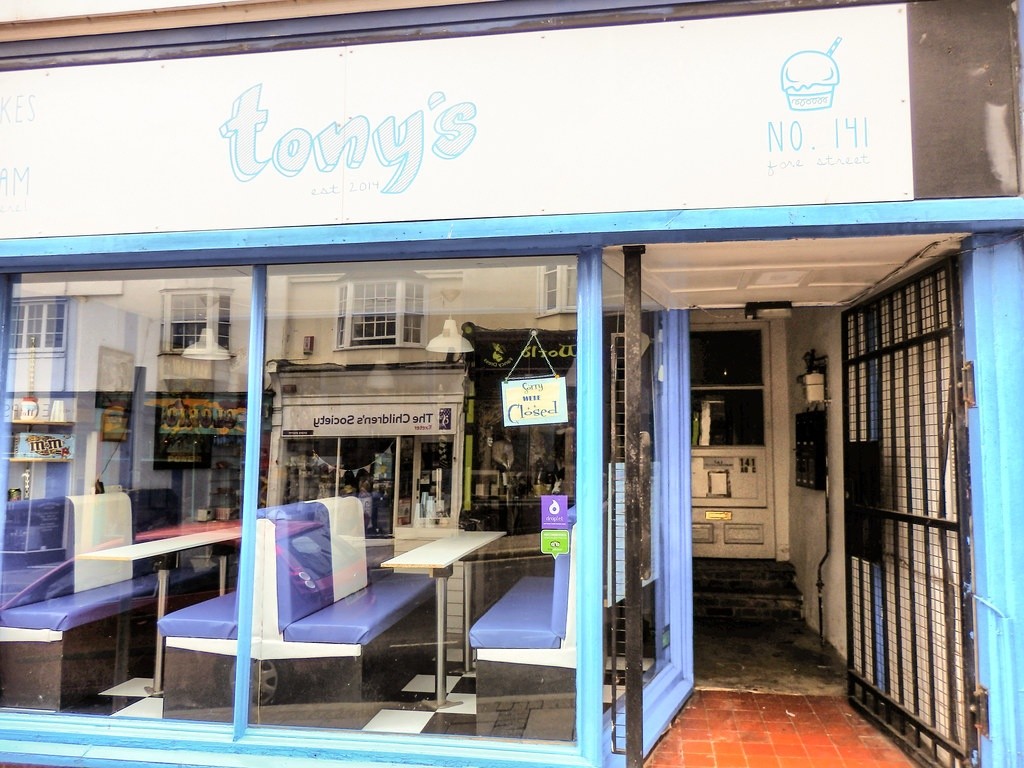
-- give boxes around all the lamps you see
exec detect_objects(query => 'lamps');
[425,314,474,353]
[180,327,229,360]
[746,301,792,320]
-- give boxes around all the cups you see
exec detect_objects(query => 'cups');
[413,492,437,525]
[533,484,551,497]
[9,488,22,501]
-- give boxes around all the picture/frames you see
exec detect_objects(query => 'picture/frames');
[95,346,134,412]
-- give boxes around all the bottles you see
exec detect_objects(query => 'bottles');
[536,467,553,484]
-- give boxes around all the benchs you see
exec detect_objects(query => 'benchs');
[469,504,576,741]
[157,495,437,730]
[0,490,218,712]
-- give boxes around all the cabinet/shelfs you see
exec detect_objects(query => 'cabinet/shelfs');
[10,419,73,463]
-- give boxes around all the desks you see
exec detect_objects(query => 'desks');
[380,531,508,709]
[76,525,241,695]
